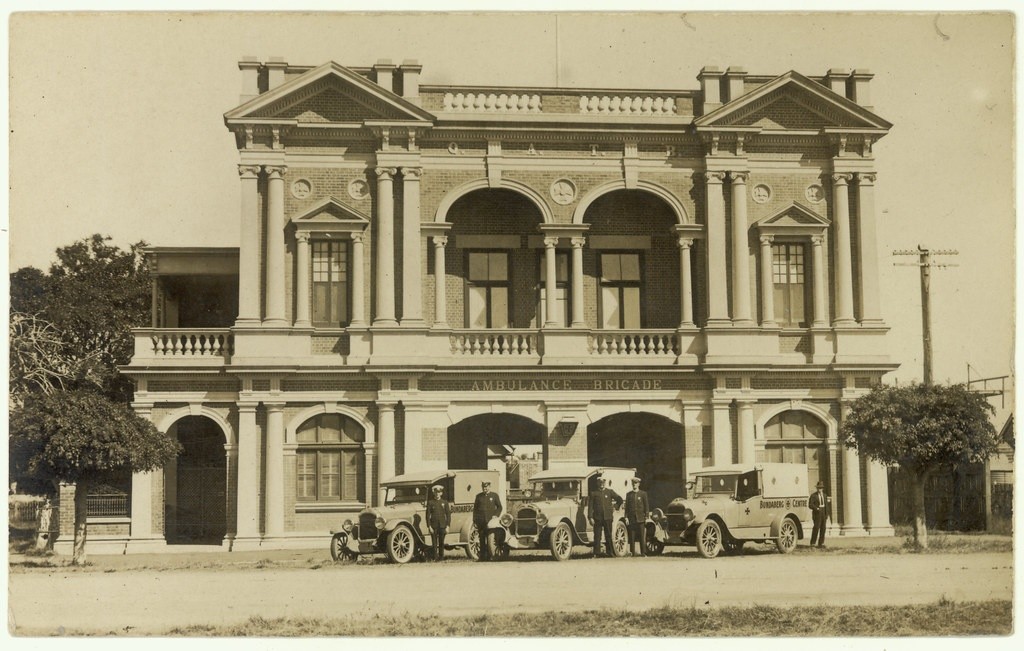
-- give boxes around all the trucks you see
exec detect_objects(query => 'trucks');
[640,460,810,560]
[485,465,638,562]
[328,468,503,564]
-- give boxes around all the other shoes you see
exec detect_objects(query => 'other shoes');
[818,544,825,548]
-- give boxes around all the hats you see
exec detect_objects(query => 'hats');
[631,478,641,482]
[814,481,826,487]
[596,477,606,482]
[482,480,490,485]
[432,485,444,493]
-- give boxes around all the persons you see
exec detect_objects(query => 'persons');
[625,477,649,557]
[587,477,623,558]
[808,481,829,547]
[473,480,503,562]
[426,485,451,561]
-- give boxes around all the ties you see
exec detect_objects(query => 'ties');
[821,493,823,505]
